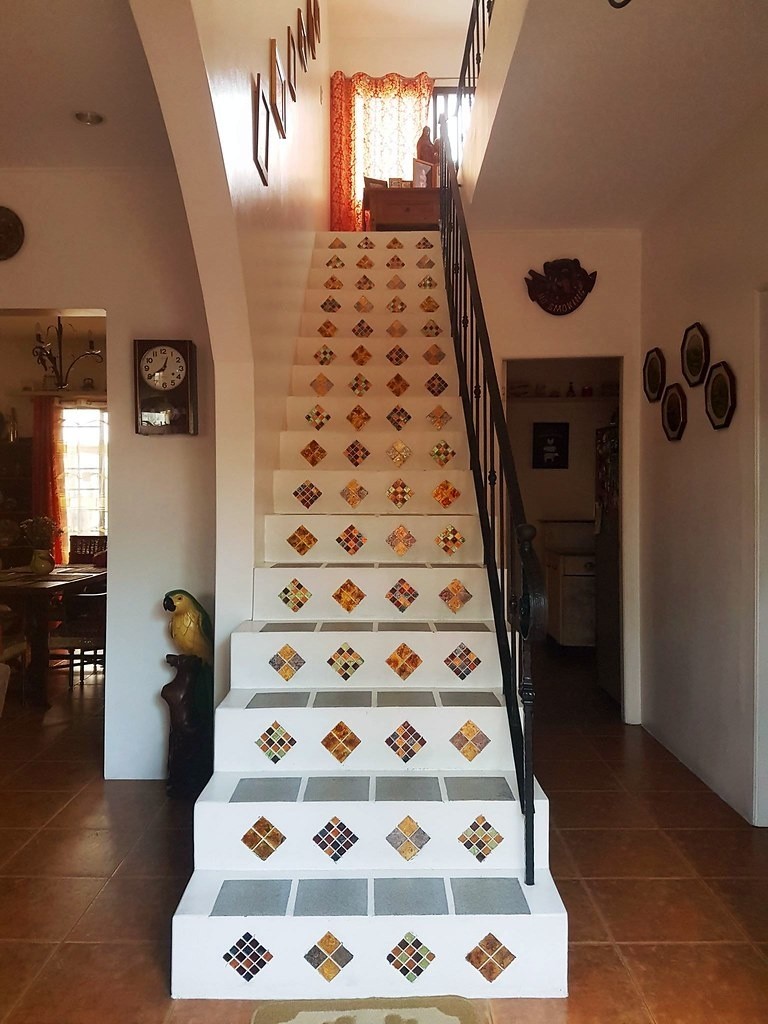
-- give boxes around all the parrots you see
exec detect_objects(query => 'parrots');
[162,589,214,666]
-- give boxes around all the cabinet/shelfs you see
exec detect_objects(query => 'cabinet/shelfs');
[367,187,440,230]
[545,549,596,647]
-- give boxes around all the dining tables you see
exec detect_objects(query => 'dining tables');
[0,564,107,706]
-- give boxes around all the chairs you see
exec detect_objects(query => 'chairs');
[0,604,28,706]
[47,584,105,691]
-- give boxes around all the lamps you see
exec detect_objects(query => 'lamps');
[33,316,103,390]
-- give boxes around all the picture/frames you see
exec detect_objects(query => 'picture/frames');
[307,0,316,59]
[314,0,321,43]
[681,322,709,387]
[255,73,271,186]
[288,26,297,102]
[705,361,736,430]
[643,347,666,403]
[270,39,288,139]
[298,8,307,72]
[661,383,687,441]
[532,422,568,469]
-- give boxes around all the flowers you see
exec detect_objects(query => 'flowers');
[19,516,64,549]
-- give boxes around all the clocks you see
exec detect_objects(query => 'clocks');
[133,339,198,435]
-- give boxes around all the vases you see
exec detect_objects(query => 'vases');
[30,549,55,576]
